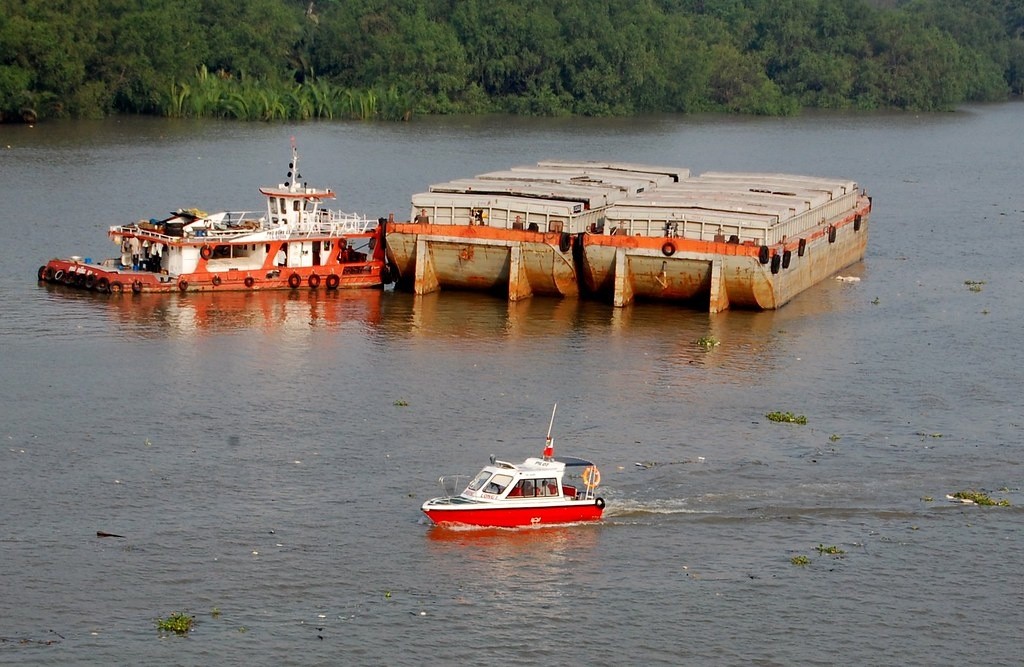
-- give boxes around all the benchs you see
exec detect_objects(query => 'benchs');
[547,483,577,500]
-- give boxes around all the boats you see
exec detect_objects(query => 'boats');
[420,404,605,529]
[37,136,387,294]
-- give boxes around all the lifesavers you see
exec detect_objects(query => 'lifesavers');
[36,264,123,295]
[199,244,214,260]
[558,233,572,252]
[244,276,255,286]
[828,225,837,243]
[131,280,143,292]
[308,273,321,288]
[326,274,340,289]
[178,279,189,290]
[212,275,222,286]
[582,465,600,490]
[660,242,675,257]
[756,237,806,275]
[854,213,863,232]
[289,272,302,288]
